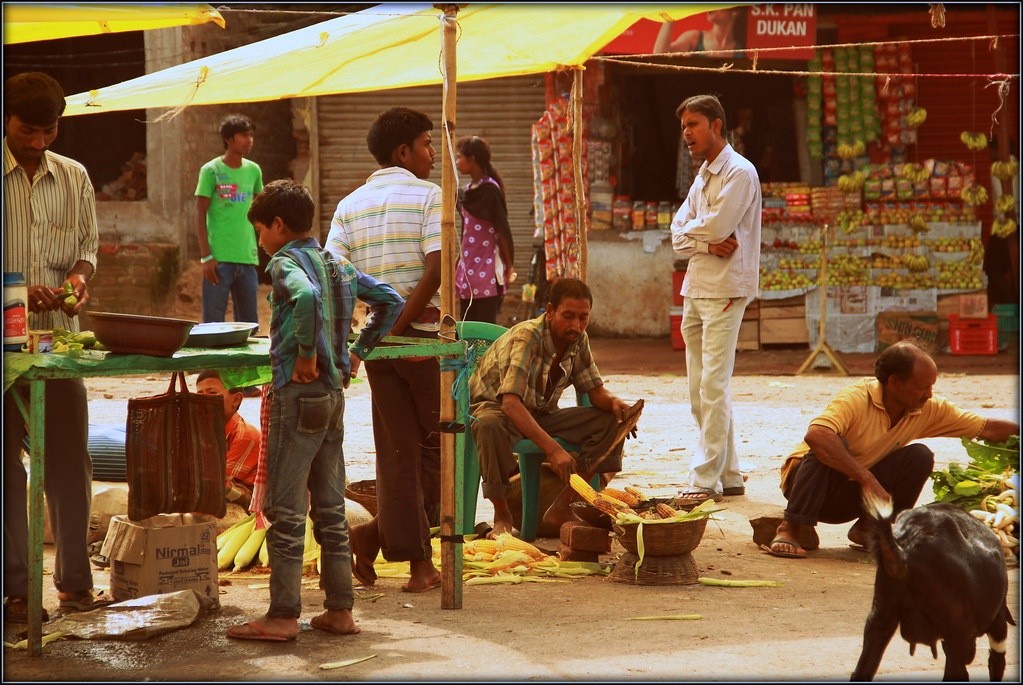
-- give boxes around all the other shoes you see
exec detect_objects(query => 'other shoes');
[4,601,52,624]
[57,587,108,610]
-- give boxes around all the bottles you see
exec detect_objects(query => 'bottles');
[3,272,28,352]
[657,202,671,229]
[613,196,631,230]
[632,201,644,230]
[589,181,613,230]
[645,202,657,230]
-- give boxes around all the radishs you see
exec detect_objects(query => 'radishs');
[969,473,1019,562]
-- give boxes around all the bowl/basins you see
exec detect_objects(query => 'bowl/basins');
[186,322,259,346]
[87,311,199,356]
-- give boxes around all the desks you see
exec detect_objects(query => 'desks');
[3,337,468,655]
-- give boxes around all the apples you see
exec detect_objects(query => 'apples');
[758,208,985,292]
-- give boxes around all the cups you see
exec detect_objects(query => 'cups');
[29,331,54,353]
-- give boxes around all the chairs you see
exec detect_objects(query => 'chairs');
[456,322,601,542]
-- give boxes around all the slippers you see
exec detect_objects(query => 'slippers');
[311,614,358,635]
[724,487,746,495]
[770,539,807,558]
[225,621,291,641]
[675,487,722,505]
[851,542,873,553]
[404,571,442,592]
[346,522,376,586]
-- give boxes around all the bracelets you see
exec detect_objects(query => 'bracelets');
[200,253,213,264]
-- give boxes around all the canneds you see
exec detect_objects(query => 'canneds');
[27,330,53,354]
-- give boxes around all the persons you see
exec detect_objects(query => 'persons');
[452,135,515,323]
[769,341,1020,559]
[225,175,406,642]
[3,71,116,627]
[323,107,443,593]
[668,95,761,501]
[653,6,748,60]
[467,279,637,543]
[196,369,263,516]
[196,113,264,397]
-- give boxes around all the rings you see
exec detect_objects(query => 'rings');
[37,299,43,305]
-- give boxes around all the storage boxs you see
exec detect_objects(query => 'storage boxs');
[100,514,221,609]
[953,314,998,355]
[670,308,686,349]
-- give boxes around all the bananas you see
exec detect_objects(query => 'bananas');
[902,254,930,272]
[840,214,857,234]
[838,170,865,193]
[960,131,1017,237]
[903,163,929,182]
[905,107,927,126]
[909,213,932,234]
[837,140,859,159]
[968,237,984,265]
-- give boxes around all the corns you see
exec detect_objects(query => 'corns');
[430,536,545,560]
[569,473,682,519]
[215,513,323,574]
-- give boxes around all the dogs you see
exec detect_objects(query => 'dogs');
[847,504,1017,682]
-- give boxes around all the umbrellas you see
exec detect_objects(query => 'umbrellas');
[3,3,228,46]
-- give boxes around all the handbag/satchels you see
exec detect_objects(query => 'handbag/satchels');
[124,373,235,523]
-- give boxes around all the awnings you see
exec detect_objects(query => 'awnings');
[59,3,737,120]
